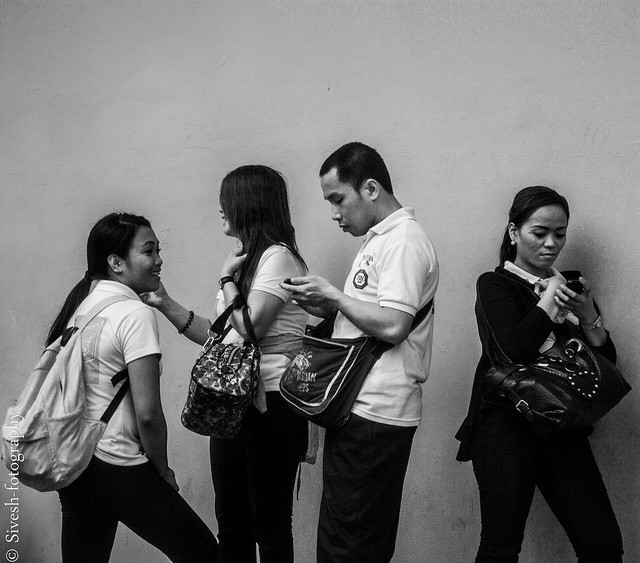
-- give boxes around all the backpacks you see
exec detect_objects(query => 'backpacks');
[2,294,138,492]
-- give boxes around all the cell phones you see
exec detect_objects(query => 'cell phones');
[285,279,300,295]
[559,270,583,295]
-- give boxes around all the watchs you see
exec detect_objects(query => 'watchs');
[218,275,234,290]
[582,315,603,335]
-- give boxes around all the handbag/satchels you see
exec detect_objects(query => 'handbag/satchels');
[279,320,383,431]
[181,303,262,440]
[477,272,632,442]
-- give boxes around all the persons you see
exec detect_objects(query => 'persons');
[140,165,307,561]
[280,142,439,562]
[43,212,218,561]
[455,184,625,563]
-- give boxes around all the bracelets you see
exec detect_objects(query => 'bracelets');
[178,310,195,335]
